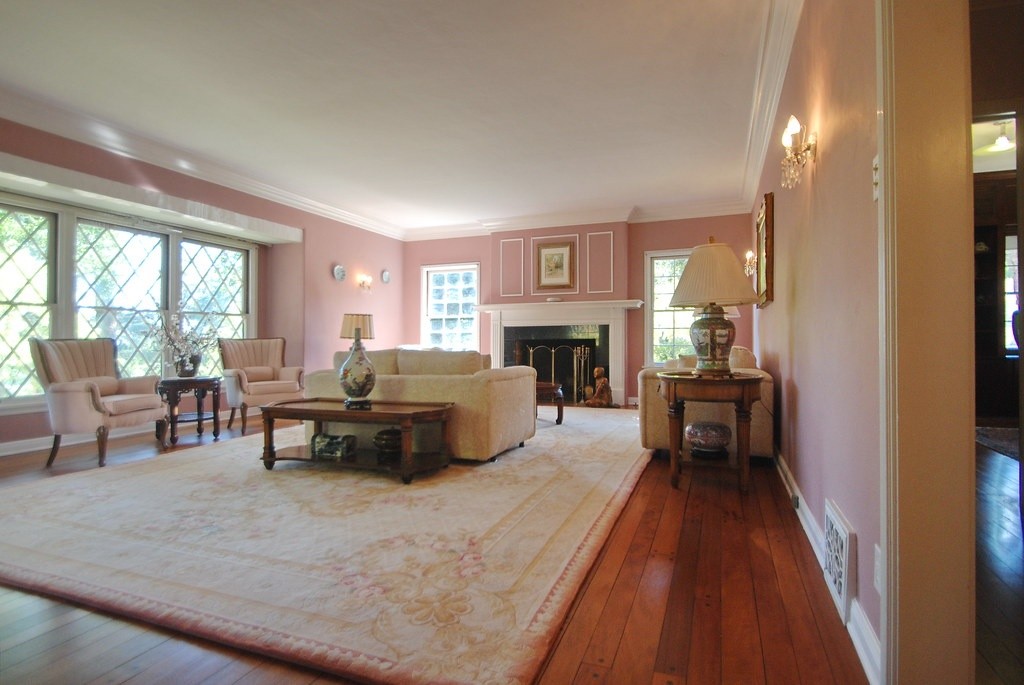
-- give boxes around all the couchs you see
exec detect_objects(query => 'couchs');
[306,334,538,464]
[638,343,771,459]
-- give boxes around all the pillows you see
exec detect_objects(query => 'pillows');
[483,351,492,368]
[677,352,696,368]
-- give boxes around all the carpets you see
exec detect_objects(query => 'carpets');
[0,399,656,685]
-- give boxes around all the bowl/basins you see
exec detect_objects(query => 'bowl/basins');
[372,427,401,452]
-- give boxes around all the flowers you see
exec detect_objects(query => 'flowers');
[136,299,222,374]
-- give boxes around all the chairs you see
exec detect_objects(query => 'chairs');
[215,334,305,434]
[26,336,173,464]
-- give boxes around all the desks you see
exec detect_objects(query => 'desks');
[253,398,456,483]
[155,374,222,442]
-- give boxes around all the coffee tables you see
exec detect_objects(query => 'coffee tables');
[534,380,564,425]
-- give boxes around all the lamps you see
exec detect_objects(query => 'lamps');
[355,273,373,291]
[744,249,757,277]
[338,312,376,351]
[779,112,817,191]
[670,233,761,378]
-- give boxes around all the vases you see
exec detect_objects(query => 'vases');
[177,349,202,377]
[341,327,377,396]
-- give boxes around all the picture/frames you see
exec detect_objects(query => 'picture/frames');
[755,191,777,309]
[536,242,574,290]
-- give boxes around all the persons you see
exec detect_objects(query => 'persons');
[584,367,612,408]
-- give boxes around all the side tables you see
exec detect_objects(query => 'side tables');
[656,370,762,496]
